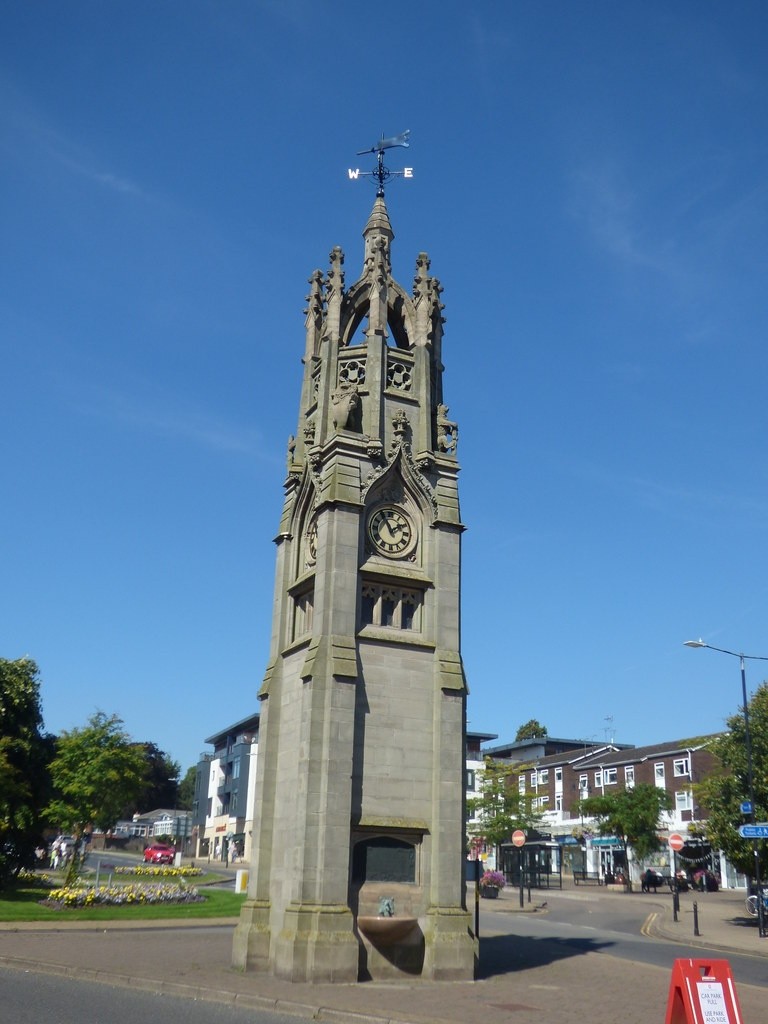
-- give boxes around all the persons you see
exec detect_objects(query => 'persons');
[676,868,718,892]
[49,838,67,870]
[216,843,221,861]
[645,870,657,893]
[230,842,237,863]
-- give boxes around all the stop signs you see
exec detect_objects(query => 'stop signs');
[668,832,685,851]
[512,829,525,848]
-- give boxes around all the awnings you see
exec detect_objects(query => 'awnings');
[590,835,626,846]
[663,830,710,847]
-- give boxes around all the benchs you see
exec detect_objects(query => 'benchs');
[573,870,604,886]
[607,883,628,892]
[642,876,674,893]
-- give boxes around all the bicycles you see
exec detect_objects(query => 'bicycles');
[746,888,768,917]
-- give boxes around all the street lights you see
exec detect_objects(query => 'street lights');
[682,640,768,937]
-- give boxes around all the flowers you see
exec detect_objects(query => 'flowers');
[479,870,506,888]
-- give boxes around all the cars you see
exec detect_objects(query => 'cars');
[143,842,175,864]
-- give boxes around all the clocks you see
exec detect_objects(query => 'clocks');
[365,502,419,560]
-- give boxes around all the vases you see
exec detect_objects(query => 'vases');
[480,884,499,899]
